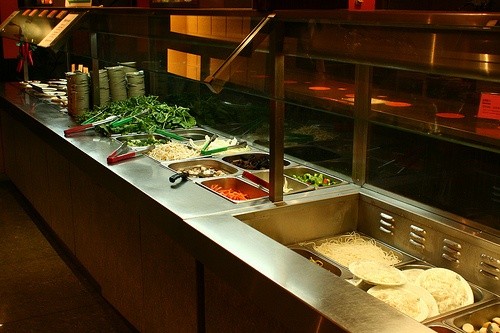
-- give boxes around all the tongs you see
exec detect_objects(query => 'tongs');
[64,115,118,135]
[107,141,156,164]
[242,170,270,188]
[201,135,248,154]
[81,107,123,125]
[155,129,195,141]
[109,108,152,127]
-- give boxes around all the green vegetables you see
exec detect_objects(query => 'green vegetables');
[73,95,197,137]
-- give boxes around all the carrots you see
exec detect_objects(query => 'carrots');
[210,183,250,200]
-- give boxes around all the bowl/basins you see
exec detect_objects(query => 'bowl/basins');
[65,61,144,116]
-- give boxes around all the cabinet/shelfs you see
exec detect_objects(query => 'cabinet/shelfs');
[0,107,350,333]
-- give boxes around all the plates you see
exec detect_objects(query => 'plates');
[20,79,67,112]
[365,285,428,322]
[401,269,424,282]
[349,262,406,286]
[238,70,464,121]
[416,268,474,314]
[402,284,438,318]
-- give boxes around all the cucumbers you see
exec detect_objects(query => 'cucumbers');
[291,173,340,187]
